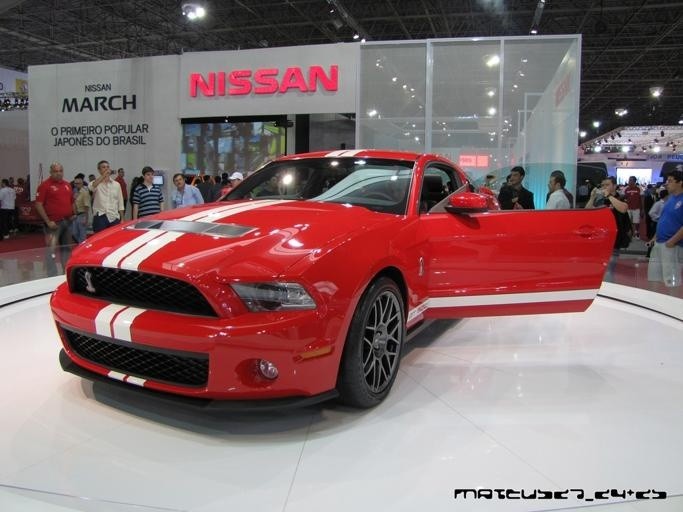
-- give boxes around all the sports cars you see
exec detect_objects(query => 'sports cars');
[48,143,621,418]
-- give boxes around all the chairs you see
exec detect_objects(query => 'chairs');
[421,176,448,212]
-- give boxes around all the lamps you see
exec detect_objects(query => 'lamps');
[642,131,665,152]
[666,142,677,152]
[530,23,538,34]
[0,98,28,112]
[598,132,622,145]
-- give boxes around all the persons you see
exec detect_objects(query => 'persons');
[478,166,683,299]
[0,161,316,278]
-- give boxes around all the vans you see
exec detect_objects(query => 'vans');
[472,161,608,208]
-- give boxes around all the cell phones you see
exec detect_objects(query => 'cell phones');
[595,188,602,196]
[112,169,116,174]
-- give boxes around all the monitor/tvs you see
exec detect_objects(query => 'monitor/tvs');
[152,175,164,188]
